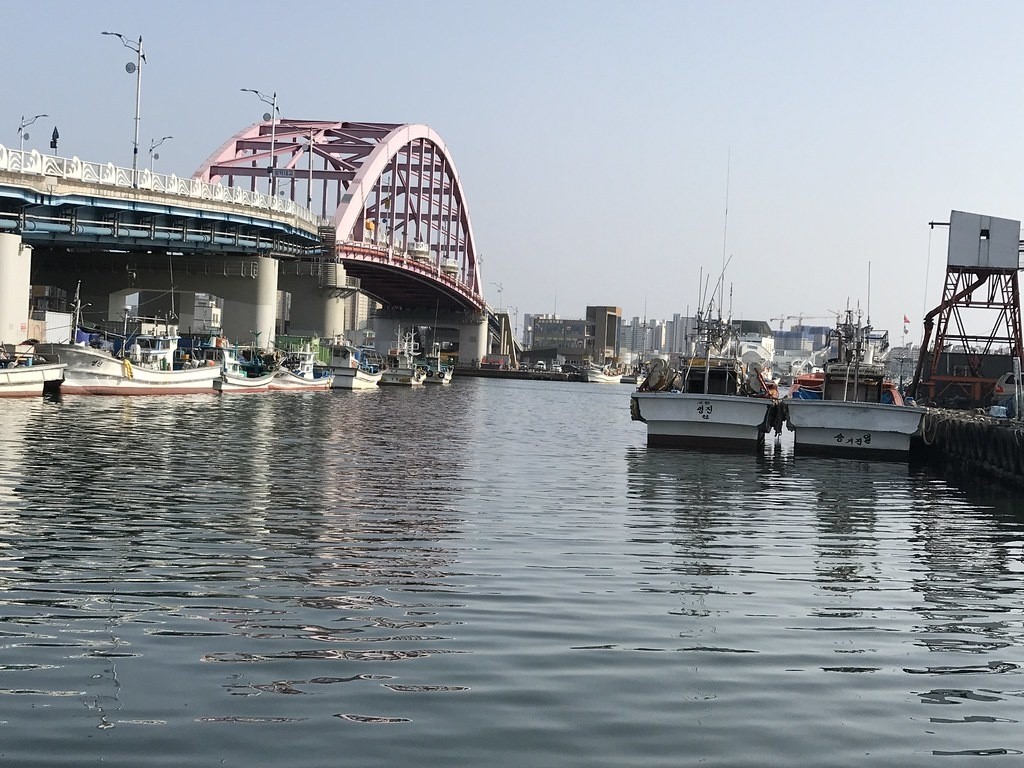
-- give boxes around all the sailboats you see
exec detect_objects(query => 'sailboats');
[635,299,652,386]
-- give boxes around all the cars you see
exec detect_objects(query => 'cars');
[550,364,562,372]
[535,361,546,372]
[518,362,530,371]
[561,364,581,373]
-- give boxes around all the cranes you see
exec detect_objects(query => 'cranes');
[786,312,837,332]
[770,314,788,331]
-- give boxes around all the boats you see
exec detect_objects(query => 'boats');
[579,360,623,383]
[0,297,455,399]
[630,145,777,447]
[782,260,931,458]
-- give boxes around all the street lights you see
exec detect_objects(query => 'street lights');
[149,135,174,173]
[240,87,282,197]
[101,31,147,189]
[16,114,49,173]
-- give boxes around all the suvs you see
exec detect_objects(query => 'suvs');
[991,372,1024,413]
